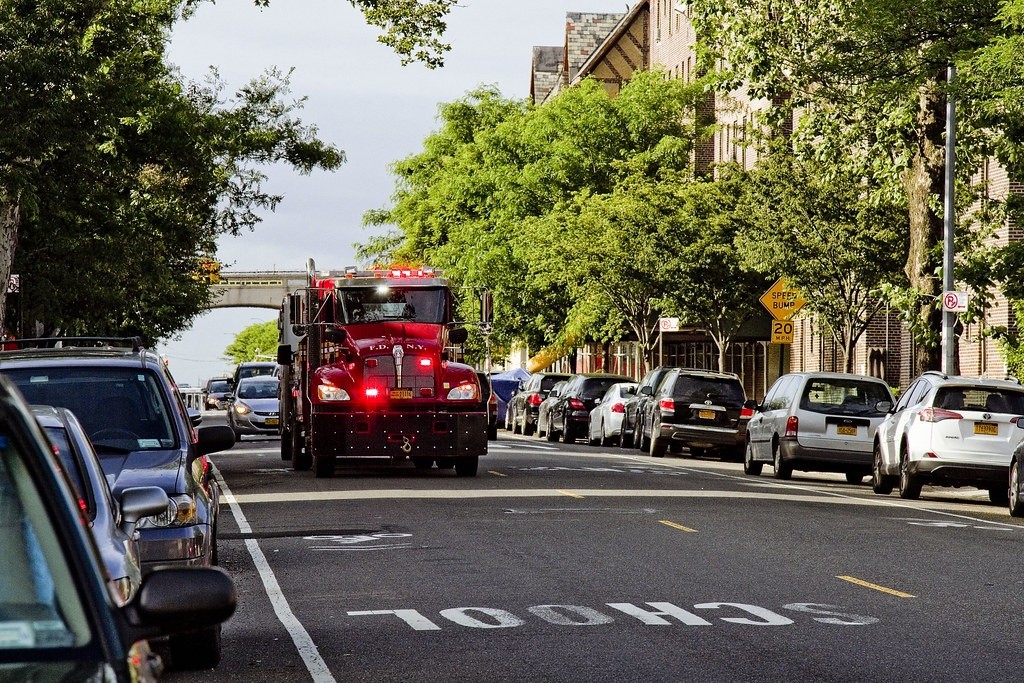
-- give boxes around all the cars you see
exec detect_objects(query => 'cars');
[1008,416,1024,518]
[587,382,640,447]
[505,372,576,438]
[0,373,238,683]
[11,403,171,613]
[166,361,282,442]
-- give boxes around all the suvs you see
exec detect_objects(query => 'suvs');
[638,367,754,462]
[619,364,679,450]
[0,332,238,671]
[545,372,638,444]
[871,370,1024,506]
[744,371,899,485]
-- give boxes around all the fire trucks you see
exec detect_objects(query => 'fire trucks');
[276,258,494,480]
